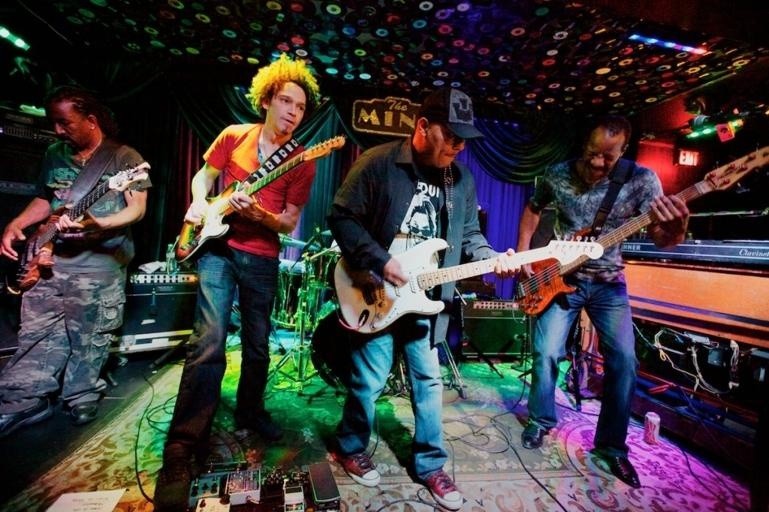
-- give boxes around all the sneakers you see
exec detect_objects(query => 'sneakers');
[344,451,381,487]
[70,400,98,427]
[0,398,52,439]
[423,469,464,509]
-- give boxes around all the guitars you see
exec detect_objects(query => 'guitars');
[174,135,347,267]
[515,144,769,318]
[332,234,604,334]
[5,161,152,294]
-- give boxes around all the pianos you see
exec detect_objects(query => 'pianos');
[618,235,765,265]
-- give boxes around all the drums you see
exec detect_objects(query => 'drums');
[271,259,313,331]
[302,251,340,324]
[307,299,353,394]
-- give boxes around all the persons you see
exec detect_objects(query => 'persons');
[163,54,322,468]
[0,84,153,441]
[515,114,688,488]
[324,89,522,510]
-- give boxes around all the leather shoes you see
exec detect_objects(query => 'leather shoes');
[521,425,546,449]
[154,446,205,511]
[247,412,282,442]
[597,449,641,489]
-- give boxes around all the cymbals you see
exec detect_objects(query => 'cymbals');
[278,233,319,252]
[319,229,332,236]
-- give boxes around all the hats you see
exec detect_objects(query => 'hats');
[421,88,485,139]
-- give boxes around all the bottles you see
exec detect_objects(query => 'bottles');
[644,412,661,446]
[165,244,177,274]
